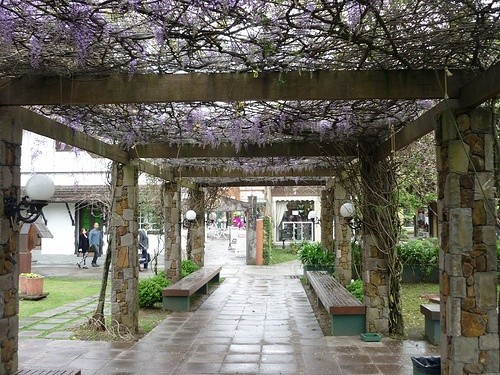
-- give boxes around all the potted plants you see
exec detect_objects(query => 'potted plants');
[298,242,327,273]
[19,272,44,295]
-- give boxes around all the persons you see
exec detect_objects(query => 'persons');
[138,224,149,269]
[87,222,102,267]
[76,227,89,270]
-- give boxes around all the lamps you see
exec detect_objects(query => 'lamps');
[204,212,216,226]
[340,203,363,232]
[4,175,55,233]
[309,211,322,227]
[178,210,197,228]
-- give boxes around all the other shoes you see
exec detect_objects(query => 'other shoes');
[77,262,81,268]
[83,265,88,268]
[91,263,96,267]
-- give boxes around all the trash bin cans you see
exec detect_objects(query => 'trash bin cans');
[411,356,441,375]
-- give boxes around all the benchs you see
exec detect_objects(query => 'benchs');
[307,271,365,336]
[421,303,441,346]
[162,265,222,311]
